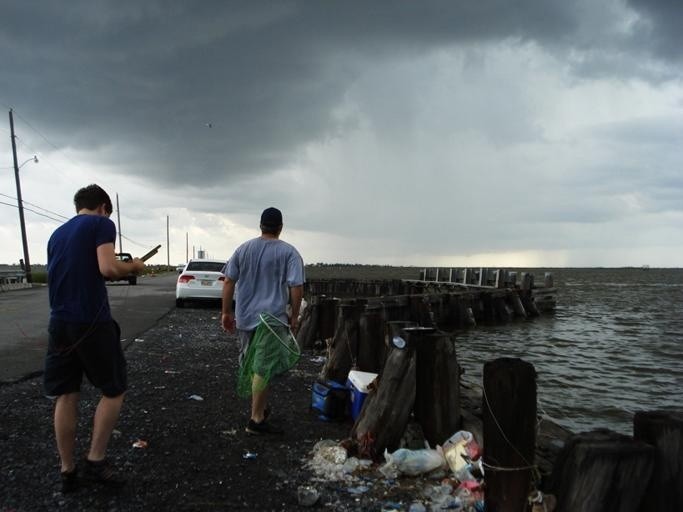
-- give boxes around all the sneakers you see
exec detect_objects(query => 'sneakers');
[58,460,127,493]
[244,405,285,436]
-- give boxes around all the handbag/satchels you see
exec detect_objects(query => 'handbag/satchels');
[311,379,347,422]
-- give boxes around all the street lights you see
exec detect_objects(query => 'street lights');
[16,156,39,282]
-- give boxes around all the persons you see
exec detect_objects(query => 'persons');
[220,207,307,435]
[46,184,147,484]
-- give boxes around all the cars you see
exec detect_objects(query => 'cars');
[115,253,137,284]
[175,259,237,308]
